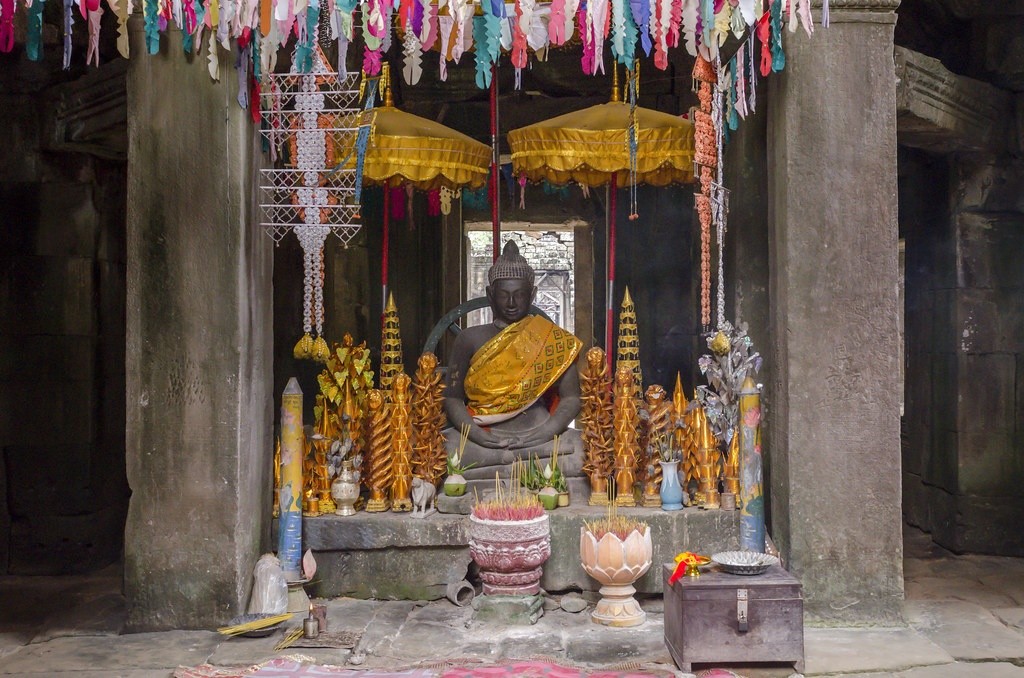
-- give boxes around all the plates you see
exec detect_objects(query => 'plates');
[711,550,778,575]
[229,613,285,637]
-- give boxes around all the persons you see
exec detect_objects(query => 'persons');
[420,239,592,479]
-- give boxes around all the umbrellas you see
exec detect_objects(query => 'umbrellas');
[334,87,492,389]
[506,60,702,407]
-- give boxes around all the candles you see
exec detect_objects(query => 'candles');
[309,603,313,620]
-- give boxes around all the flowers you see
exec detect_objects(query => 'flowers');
[311,413,363,482]
[638,400,697,485]
[446,448,482,475]
[518,453,568,493]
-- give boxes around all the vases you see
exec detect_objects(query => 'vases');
[330,461,360,516]
[444,474,468,496]
[658,458,684,511]
[558,490,569,507]
[536,486,559,510]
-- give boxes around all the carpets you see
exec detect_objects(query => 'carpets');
[171,653,747,678]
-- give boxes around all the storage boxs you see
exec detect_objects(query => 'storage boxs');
[663,562,806,675]
